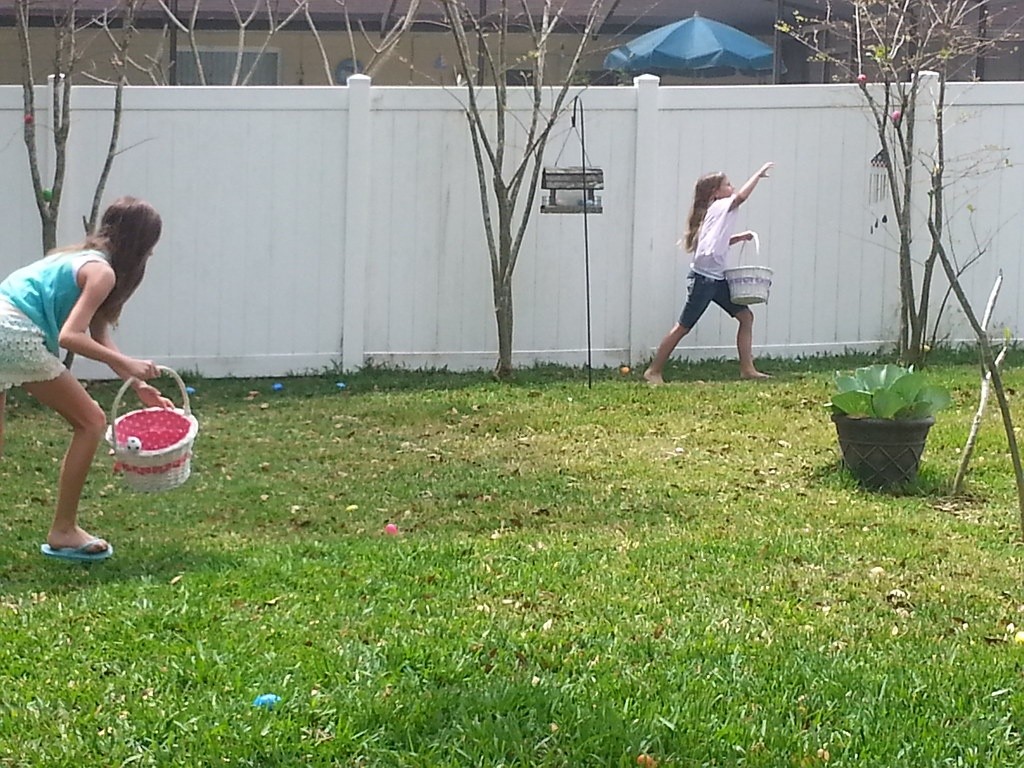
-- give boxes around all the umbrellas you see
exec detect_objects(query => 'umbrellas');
[603,10,788,84]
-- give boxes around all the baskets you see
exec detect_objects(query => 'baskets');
[104,365,199,494]
[723,231,774,305]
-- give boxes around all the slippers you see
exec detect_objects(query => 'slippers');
[40,538,113,561]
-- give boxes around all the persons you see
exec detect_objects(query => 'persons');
[0,195,174,559]
[643,161,775,385]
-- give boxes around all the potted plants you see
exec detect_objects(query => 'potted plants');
[823,363,950,486]
